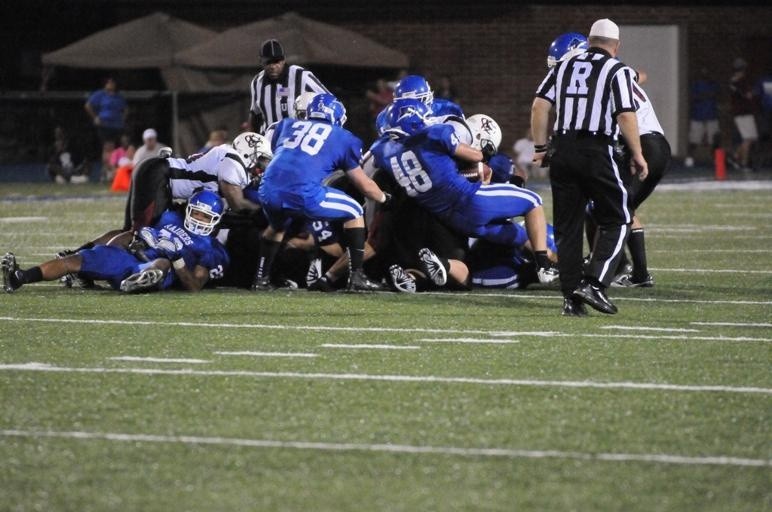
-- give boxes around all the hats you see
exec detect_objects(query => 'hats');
[261,39,284,65]
[589,18,620,41]
[143,128,157,141]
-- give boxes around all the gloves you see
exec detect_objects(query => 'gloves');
[158,237,179,259]
[139,226,159,249]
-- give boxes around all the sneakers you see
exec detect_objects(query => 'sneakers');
[2,252,17,293]
[120,270,163,292]
[536,252,653,316]
[252,248,448,293]
[56,249,102,290]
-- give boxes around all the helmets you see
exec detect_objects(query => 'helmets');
[292,92,347,127]
[547,32,586,69]
[184,190,224,237]
[232,132,274,188]
[382,75,502,156]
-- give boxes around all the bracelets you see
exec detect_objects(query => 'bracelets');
[534,143,548,154]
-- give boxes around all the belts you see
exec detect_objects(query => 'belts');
[645,131,662,135]
[555,130,602,137]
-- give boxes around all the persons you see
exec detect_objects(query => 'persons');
[726,57,772,172]
[546,34,671,287]
[532,19,650,318]
[240,40,334,133]
[0,92,395,297]
[685,58,720,166]
[310,69,558,296]
[47,77,230,187]
